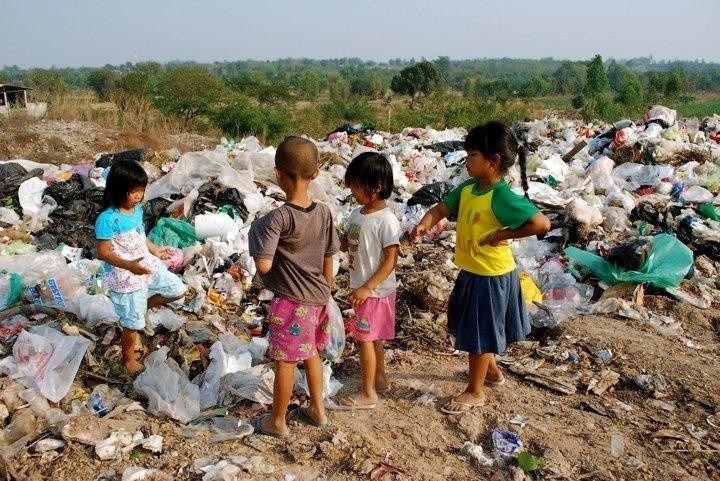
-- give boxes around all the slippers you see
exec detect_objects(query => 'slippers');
[297,406,327,429]
[143,319,155,337]
[339,384,391,411]
[250,413,285,438]
[466,370,506,386]
[440,397,485,415]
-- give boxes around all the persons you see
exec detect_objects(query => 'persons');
[408,119,551,416]
[93,159,190,374]
[336,151,401,409]
[249,136,341,441]
